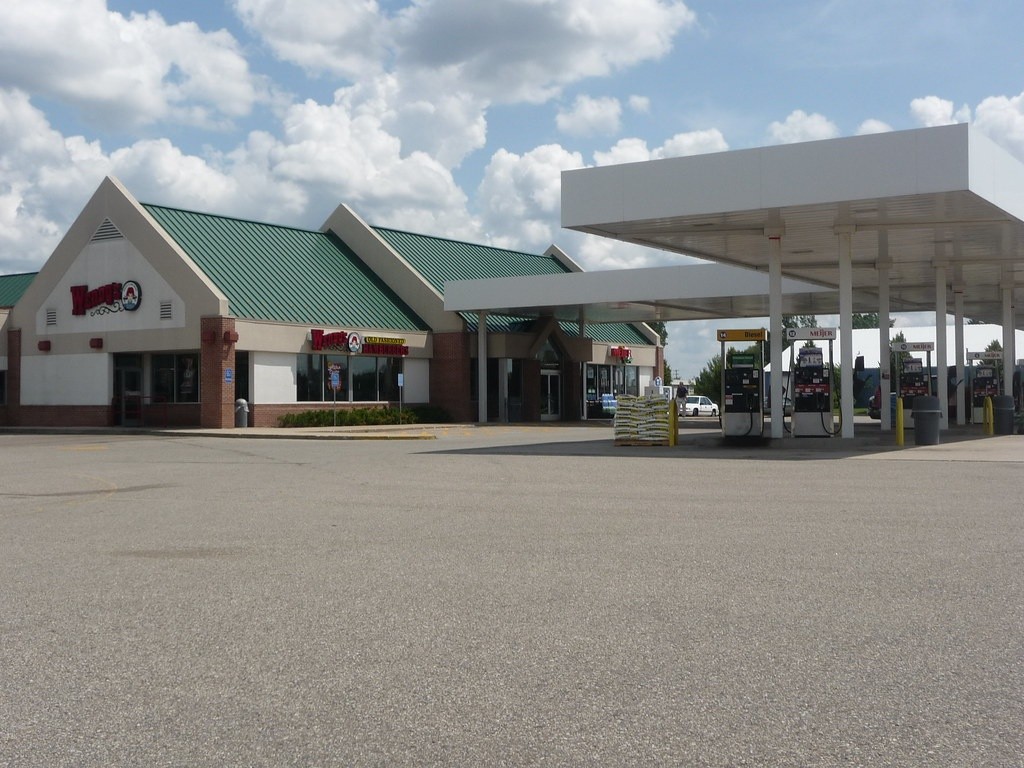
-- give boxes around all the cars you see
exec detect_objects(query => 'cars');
[679,395,719,417]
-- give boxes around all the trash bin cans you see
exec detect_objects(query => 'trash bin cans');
[992,395,1015,435]
[235,399,249,427]
[911,396,943,446]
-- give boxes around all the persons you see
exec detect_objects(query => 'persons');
[676,382,687,417]
